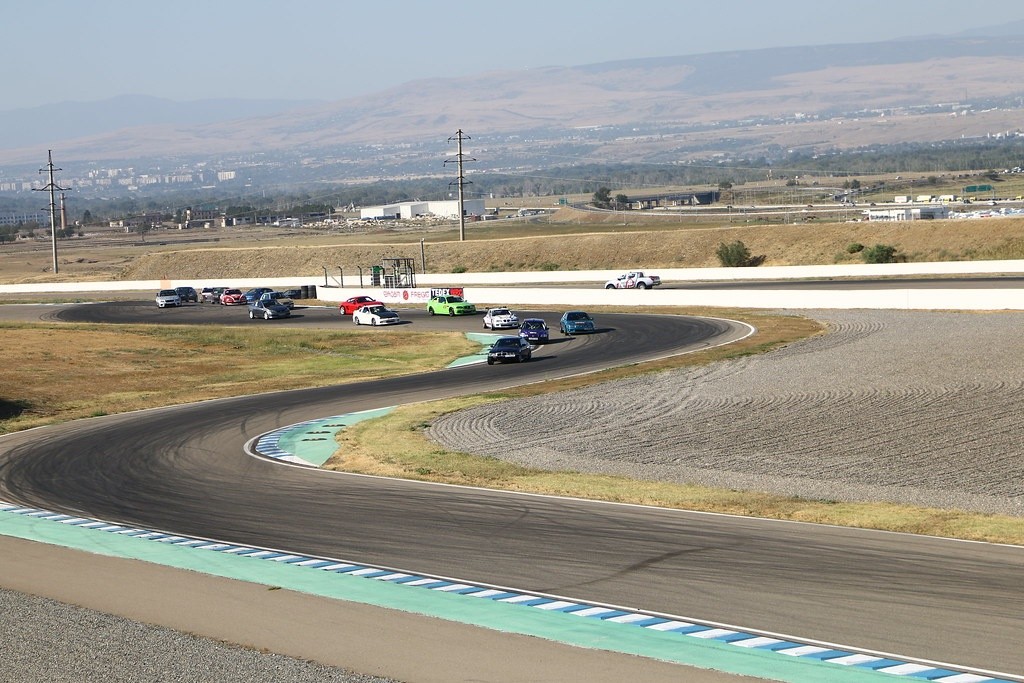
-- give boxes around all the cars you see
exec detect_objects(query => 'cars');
[338,295,384,314]
[220,287,248,306]
[242,287,274,303]
[353,305,401,328]
[155,289,183,308]
[482,307,521,332]
[426,294,477,318]
[259,291,295,310]
[487,336,532,365]
[174,286,199,304]
[211,288,229,304]
[559,311,595,334]
[248,298,292,321]
[199,287,217,304]
[518,318,550,344]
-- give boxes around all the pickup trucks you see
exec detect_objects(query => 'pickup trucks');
[604,271,662,290]
